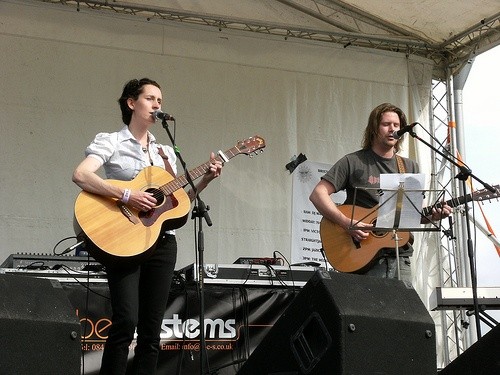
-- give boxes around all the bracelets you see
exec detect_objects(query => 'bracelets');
[120,190,131,203]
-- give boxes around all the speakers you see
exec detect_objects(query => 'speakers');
[0,273,82,374]
[235,269,437,375]
[437,324,500,375]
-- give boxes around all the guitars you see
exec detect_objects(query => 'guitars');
[72,134,266,265]
[319,183,500,275]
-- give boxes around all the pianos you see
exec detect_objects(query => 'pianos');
[429,286,500,329]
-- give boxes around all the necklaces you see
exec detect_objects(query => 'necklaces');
[142,146,148,153]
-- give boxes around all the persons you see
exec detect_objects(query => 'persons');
[308,103,451,282]
[72,78,223,375]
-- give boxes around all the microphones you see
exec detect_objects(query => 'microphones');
[152,109,175,122]
[393,122,419,139]
